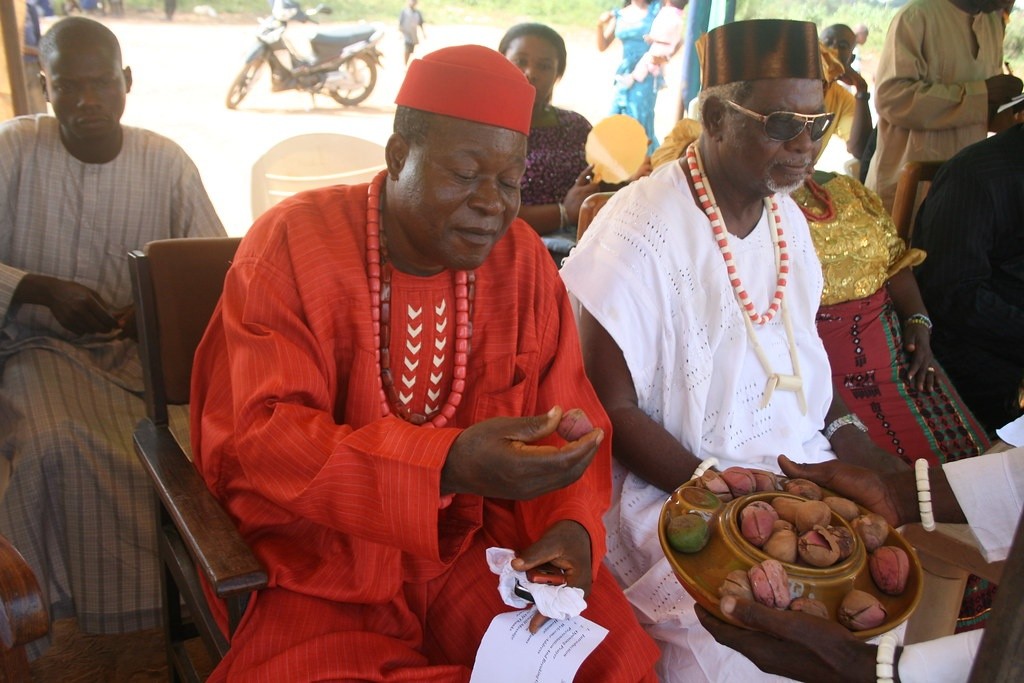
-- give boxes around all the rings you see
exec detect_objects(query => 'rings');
[927,366,935,372]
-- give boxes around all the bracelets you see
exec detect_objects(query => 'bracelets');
[559,202,568,232]
[914,458,935,531]
[855,92,870,99]
[905,313,932,329]
[690,457,719,479]
[875,631,901,683]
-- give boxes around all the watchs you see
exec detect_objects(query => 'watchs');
[824,412,869,441]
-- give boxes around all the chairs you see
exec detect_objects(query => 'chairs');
[124,236,317,683]
[246,131,394,232]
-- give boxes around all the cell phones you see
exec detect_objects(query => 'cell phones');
[514,581,537,602]
[526,564,565,585]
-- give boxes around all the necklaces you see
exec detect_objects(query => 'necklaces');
[684,140,807,414]
[368,168,472,431]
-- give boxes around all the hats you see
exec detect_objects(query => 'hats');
[398,45,535,136]
[697,19,821,89]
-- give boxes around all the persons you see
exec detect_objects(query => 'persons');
[789,170,1003,634]
[0,17,229,664]
[694,445,1024,683]
[190,46,662,683]
[597,0,688,157]
[651,23,872,176]
[398,0,427,67]
[498,24,653,236]
[912,119,1024,442]
[558,19,912,683]
[865,0,1024,239]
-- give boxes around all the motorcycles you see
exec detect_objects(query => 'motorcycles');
[225,0,386,109]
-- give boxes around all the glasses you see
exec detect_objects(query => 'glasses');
[729,100,836,143]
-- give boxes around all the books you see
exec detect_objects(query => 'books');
[996,92,1024,113]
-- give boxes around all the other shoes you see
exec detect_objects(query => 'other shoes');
[618,73,635,91]
